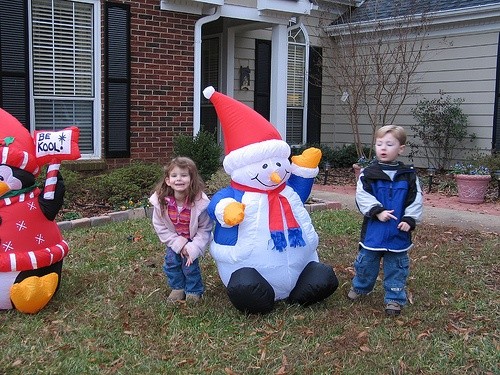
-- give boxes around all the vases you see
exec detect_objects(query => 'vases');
[454,174,491,204]
[353,163,363,181]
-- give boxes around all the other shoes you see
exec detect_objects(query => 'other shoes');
[185,292,204,305]
[166,289,184,305]
[347,286,366,300]
[386,302,402,315]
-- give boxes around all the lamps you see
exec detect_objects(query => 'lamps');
[323,164,330,185]
[426,169,435,194]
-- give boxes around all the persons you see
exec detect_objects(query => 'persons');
[149,157,214,306]
[348,125,424,310]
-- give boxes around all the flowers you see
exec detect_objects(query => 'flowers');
[356,157,372,168]
[449,162,493,175]
